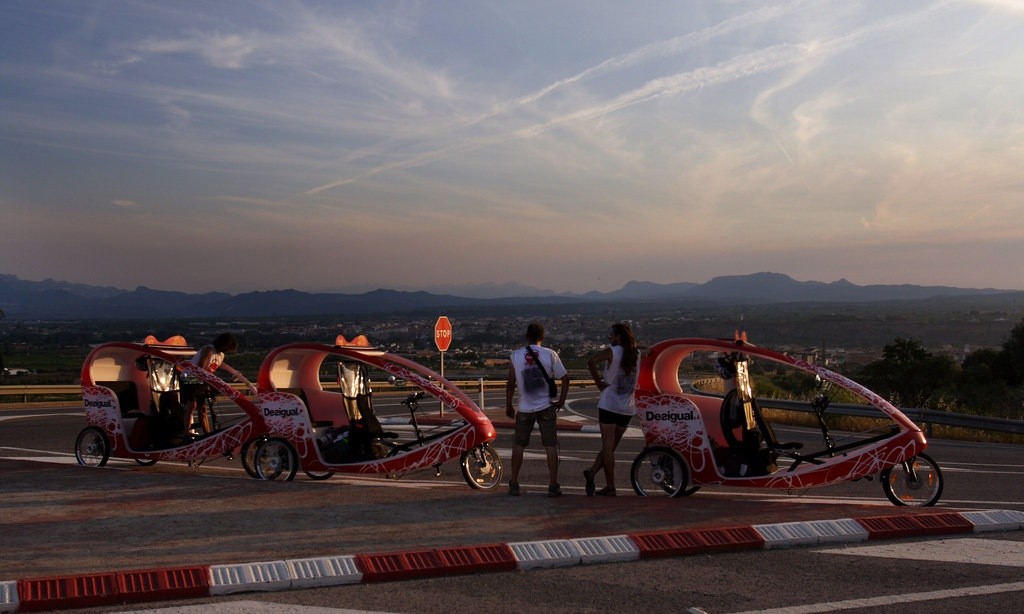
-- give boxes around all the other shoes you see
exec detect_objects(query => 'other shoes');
[508,481,520,495]
[596,487,617,497]
[549,484,562,497]
[583,468,595,497]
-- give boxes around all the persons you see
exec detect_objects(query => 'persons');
[181,332,240,441]
[583,323,641,497]
[505,322,570,496]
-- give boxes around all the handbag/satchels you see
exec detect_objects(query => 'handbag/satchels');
[548,381,557,398]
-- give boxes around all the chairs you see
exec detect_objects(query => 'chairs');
[663,392,731,466]
[148,356,188,438]
[267,389,344,464]
[95,380,152,448]
[719,359,803,463]
[338,360,399,441]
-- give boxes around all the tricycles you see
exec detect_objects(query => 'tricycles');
[629,330,944,508]
[255,334,504,493]
[74,336,286,480]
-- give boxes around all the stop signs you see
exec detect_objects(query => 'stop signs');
[434,316,454,351]
